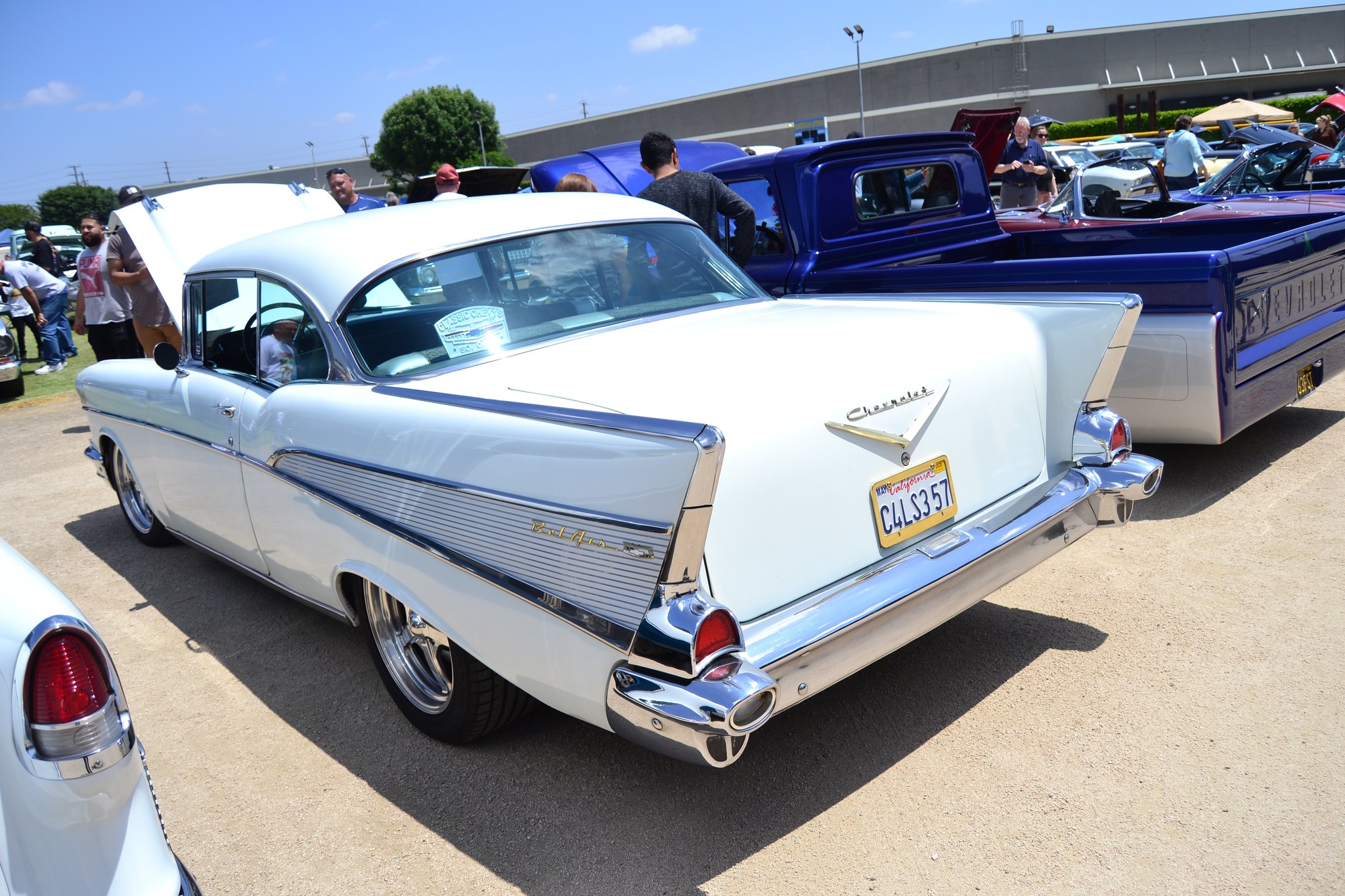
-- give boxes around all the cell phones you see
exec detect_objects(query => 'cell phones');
[1023,160,1030,165]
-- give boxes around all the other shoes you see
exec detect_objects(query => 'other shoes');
[34,362,63,374]
[40,361,68,368]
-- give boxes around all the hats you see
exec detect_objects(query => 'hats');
[17,220,41,232]
[118,185,146,205]
[435,163,459,184]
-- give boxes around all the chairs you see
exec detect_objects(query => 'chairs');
[1091,192,1124,217]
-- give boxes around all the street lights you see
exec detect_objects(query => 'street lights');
[305,141,320,189]
[841,25,867,139]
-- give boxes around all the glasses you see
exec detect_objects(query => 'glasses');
[1290,127,1298,130]
[326,168,351,179]
[1036,133,1049,138]
[1013,130,1027,134]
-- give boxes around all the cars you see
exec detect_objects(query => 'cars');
[0,538,206,896]
[0,106,1344,450]
[75,181,1170,771]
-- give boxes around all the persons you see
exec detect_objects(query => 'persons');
[1031,125,1058,206]
[0,276,44,359]
[325,167,386,214]
[626,130,756,299]
[1288,121,1304,137]
[261,319,300,384]
[18,221,78,358]
[44,235,67,279]
[1162,115,1209,191]
[1313,115,1338,149]
[0,260,68,375]
[433,163,505,302]
[73,211,145,361]
[106,185,183,357]
[904,166,930,210]
[994,116,1048,209]
[846,132,877,215]
[529,172,634,305]
[1159,128,1169,137]
[1125,133,1134,142]
[386,191,401,207]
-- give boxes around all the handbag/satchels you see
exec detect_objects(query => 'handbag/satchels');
[1157,158,1165,180]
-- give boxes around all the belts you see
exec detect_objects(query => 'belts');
[1005,181,1036,188]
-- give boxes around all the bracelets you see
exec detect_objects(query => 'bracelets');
[1033,166,1036,173]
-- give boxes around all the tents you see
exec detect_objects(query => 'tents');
[0,228,26,248]
[1190,98,1294,127]
